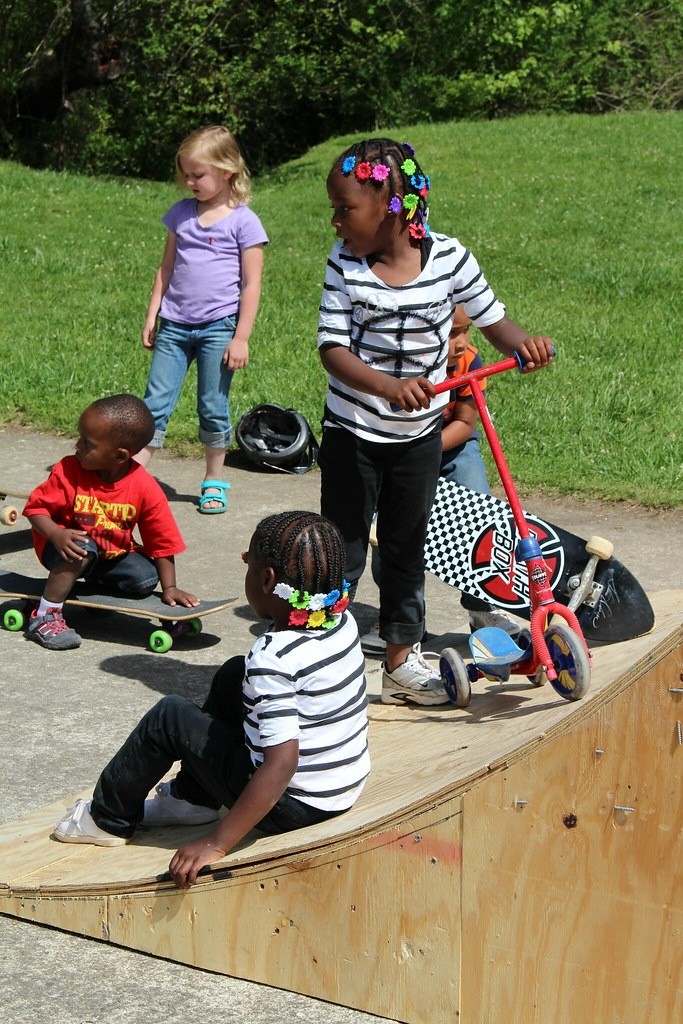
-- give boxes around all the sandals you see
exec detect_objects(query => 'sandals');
[199,480,231,514]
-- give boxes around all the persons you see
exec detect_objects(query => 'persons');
[360,302,521,655]
[21,394,201,650]
[317,138,555,705]
[131,126,270,513]
[54,510,372,886]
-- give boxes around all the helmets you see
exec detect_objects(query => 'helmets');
[234,404,310,473]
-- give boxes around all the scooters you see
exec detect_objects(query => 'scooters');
[391,339,594,706]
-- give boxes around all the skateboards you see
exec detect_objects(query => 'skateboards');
[0,568,249,657]
[359,469,657,642]
[0,483,33,528]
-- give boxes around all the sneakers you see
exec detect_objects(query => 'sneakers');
[468,609,531,642]
[54,817,133,848]
[361,618,388,655]
[141,779,219,827]
[381,641,452,705]
[28,602,82,650]
